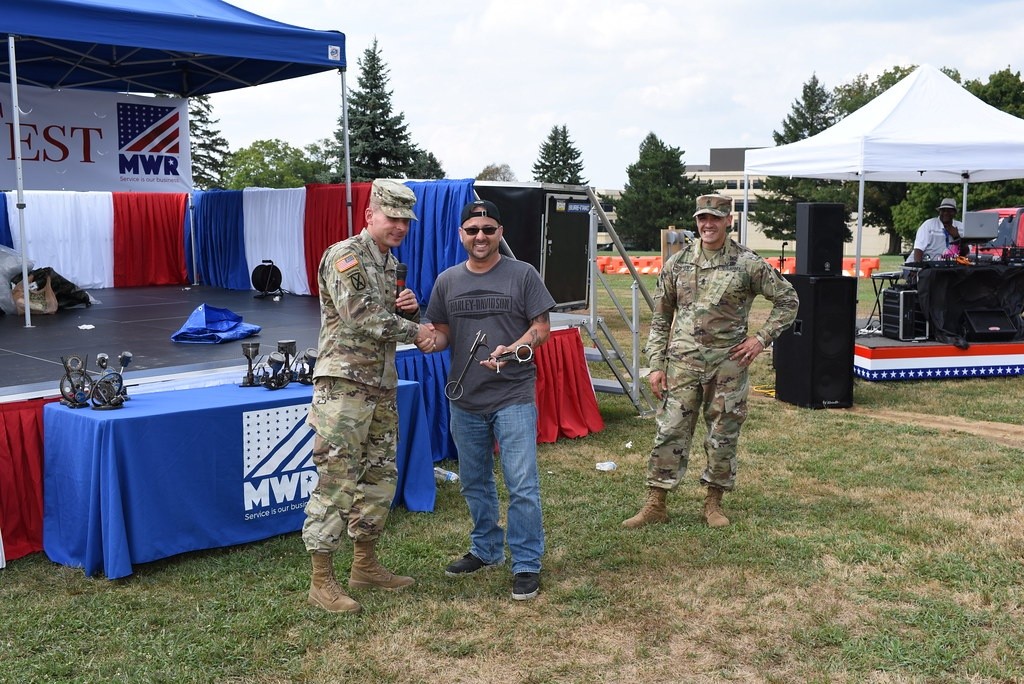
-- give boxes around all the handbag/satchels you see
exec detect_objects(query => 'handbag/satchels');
[12,274,58,317]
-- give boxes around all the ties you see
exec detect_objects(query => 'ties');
[943,229,949,247]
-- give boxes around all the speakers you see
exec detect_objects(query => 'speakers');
[773,202,858,409]
[960,309,1018,342]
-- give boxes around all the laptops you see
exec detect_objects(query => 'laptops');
[950,212,999,245]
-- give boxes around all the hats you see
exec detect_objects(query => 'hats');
[461,200,501,226]
[370,178,419,222]
[935,198,956,211]
[692,194,732,218]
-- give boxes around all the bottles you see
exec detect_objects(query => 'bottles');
[433,467,461,483]
[595,462,617,471]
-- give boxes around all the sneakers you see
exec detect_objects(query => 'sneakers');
[512,572,540,601]
[444,552,505,578]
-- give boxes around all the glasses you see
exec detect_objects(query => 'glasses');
[461,227,499,235]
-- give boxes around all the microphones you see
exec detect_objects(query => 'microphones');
[395,264,408,317]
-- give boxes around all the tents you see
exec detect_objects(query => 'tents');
[740,61,1024,305]
[0,0,352,329]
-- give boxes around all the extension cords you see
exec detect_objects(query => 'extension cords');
[859,329,881,335]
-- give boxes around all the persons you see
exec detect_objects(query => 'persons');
[412,198,556,601]
[301,176,437,614]
[903,197,970,284]
[621,194,800,532]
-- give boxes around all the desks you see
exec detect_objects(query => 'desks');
[43,371,437,580]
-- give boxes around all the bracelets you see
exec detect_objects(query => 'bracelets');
[955,237,962,241]
[755,334,767,348]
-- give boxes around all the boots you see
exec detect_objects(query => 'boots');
[704,487,730,528]
[349,539,416,591]
[621,487,671,529]
[306,551,362,615]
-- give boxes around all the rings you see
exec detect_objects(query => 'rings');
[433,344,436,349]
[746,353,750,359]
